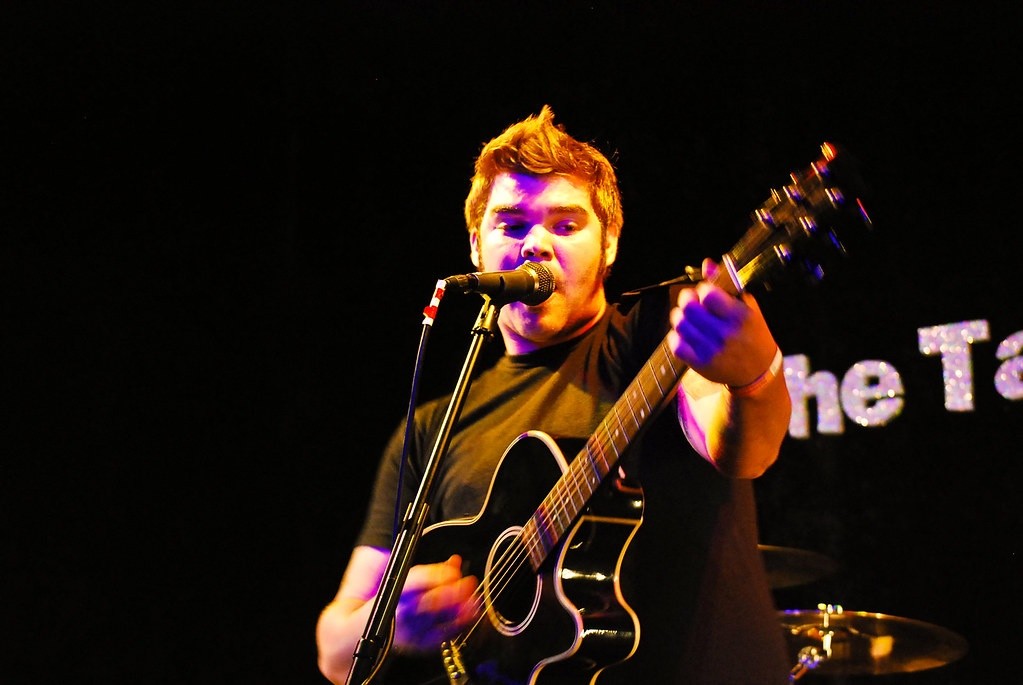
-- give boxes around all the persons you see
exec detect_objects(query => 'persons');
[315,103,795,685]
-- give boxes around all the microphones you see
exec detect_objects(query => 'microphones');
[444,260,557,307]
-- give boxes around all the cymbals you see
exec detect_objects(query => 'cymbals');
[773,607,970,680]
[753,539,837,589]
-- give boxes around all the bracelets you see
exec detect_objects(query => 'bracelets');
[724,347,783,397]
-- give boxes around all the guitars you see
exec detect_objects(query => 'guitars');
[368,135,875,684]
